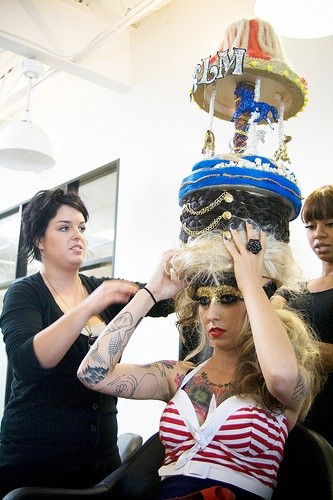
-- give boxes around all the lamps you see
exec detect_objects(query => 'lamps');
[0,58,55,171]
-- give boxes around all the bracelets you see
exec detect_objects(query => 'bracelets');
[141,285,158,304]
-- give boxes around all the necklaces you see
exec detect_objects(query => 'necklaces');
[42,267,95,349]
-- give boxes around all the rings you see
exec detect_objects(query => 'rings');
[246,238,262,254]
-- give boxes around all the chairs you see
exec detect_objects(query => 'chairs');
[2,424,333,500]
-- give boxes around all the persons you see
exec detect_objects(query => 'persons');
[275,183,333,449]
[0,189,177,500]
[76,219,311,500]
